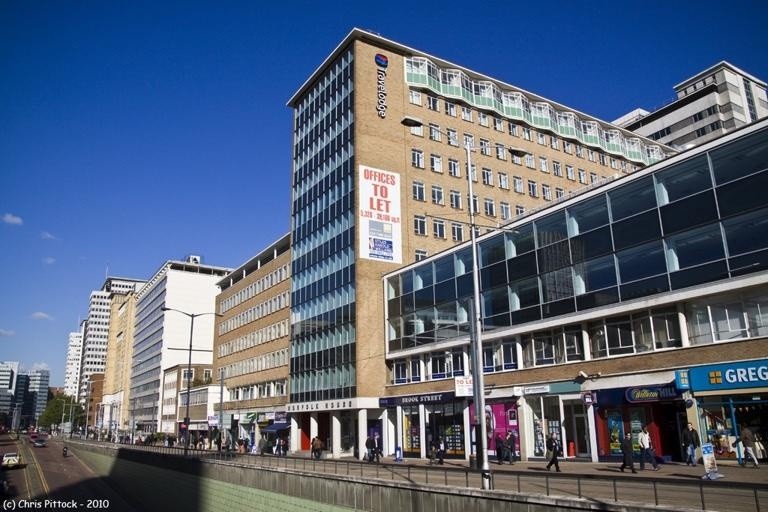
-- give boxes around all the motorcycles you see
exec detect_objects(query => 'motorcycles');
[63,447,67,458]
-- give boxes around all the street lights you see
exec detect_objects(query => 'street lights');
[85,380,96,440]
[161,308,224,456]
[400,116,527,489]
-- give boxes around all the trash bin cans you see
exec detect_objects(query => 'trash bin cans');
[469,454,477,468]
[395,447,402,461]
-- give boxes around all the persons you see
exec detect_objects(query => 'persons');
[680,422,701,466]
[731,423,761,469]
[506,429,514,454]
[224,436,231,450]
[313,436,323,458]
[495,432,505,465]
[256,435,266,455]
[238,435,243,451]
[372,432,384,462]
[619,431,638,473]
[429,438,437,462]
[310,438,316,459]
[545,432,562,472]
[365,434,375,462]
[436,435,450,464]
[637,425,659,471]
[502,434,515,465]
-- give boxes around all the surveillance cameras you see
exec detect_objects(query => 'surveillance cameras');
[578,370,588,380]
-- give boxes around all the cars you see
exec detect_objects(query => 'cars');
[29,433,46,447]
[2,452,19,468]
